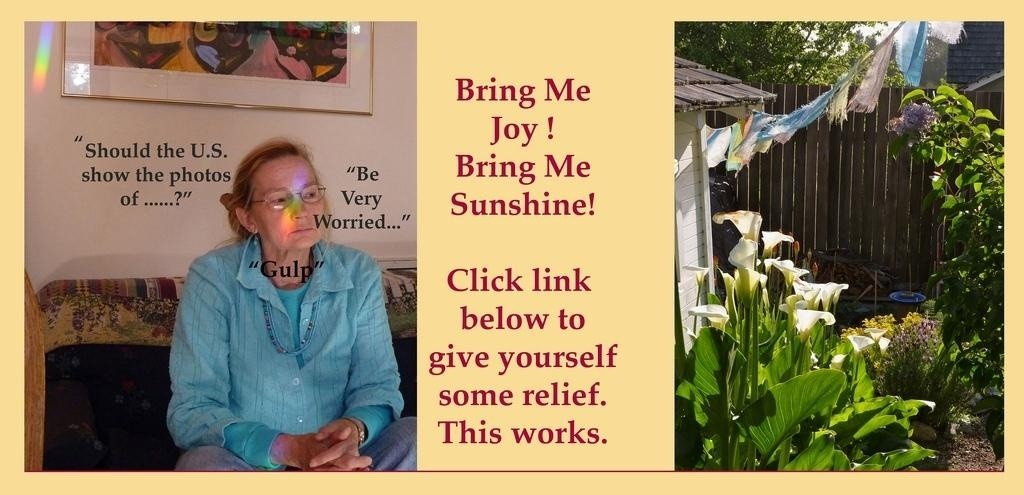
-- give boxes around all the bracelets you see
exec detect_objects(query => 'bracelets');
[341,415,366,448]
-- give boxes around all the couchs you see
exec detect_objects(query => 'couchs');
[32,266,418,472]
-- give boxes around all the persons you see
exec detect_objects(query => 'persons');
[164,136,416,473]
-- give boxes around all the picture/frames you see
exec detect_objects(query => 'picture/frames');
[61,21,376,116]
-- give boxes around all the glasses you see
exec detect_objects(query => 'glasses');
[250,183,326,209]
